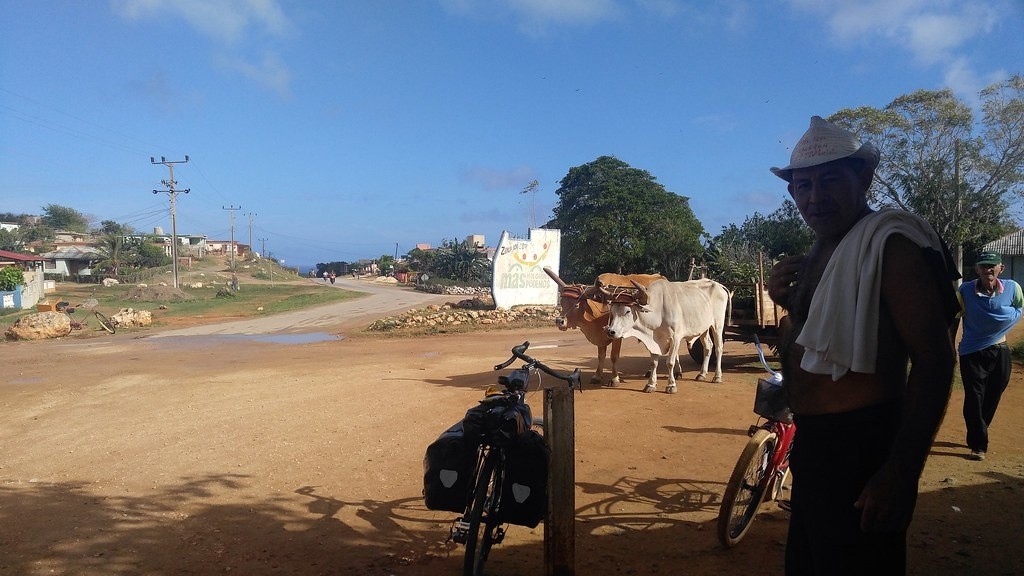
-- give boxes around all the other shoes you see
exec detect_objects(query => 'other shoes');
[972,449,985,460]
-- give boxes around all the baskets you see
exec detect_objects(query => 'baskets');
[754,378,793,424]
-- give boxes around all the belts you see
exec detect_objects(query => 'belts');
[989,342,1008,349]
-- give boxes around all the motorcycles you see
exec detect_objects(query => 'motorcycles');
[308,273,336,284]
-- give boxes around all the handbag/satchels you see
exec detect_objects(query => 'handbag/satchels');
[464,395,532,448]
[500,431,551,528]
[423,420,477,513]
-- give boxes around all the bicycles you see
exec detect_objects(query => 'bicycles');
[55,288,116,335]
[418,339,584,576]
[718,333,805,550]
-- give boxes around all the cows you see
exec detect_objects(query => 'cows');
[543,267,732,396]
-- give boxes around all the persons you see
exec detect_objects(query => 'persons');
[768,116,956,576]
[352,271,356,280]
[322,270,337,280]
[310,268,314,273]
[231,273,238,292]
[951,251,1024,461]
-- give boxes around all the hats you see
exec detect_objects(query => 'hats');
[769,116,881,181]
[976,251,1002,265]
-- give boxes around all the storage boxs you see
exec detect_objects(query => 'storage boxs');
[37,297,62,312]
